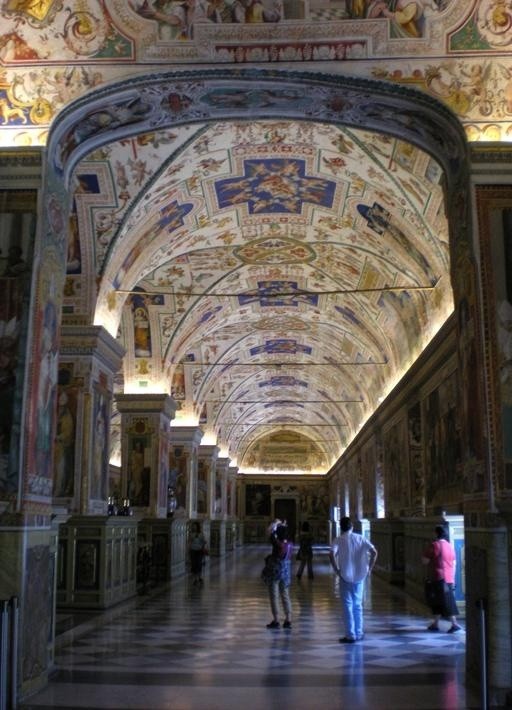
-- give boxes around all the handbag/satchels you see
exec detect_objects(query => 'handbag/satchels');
[415,559,437,580]
[201,547,209,556]
[262,553,281,585]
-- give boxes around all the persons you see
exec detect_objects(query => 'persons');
[266,521,292,629]
[136,538,170,589]
[25,530,53,677]
[295,522,315,580]
[189,522,205,585]
[422,527,462,633]
[329,518,378,642]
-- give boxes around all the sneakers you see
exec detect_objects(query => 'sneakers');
[447,625,461,633]
[428,624,439,631]
[339,637,355,643]
[266,620,291,628]
